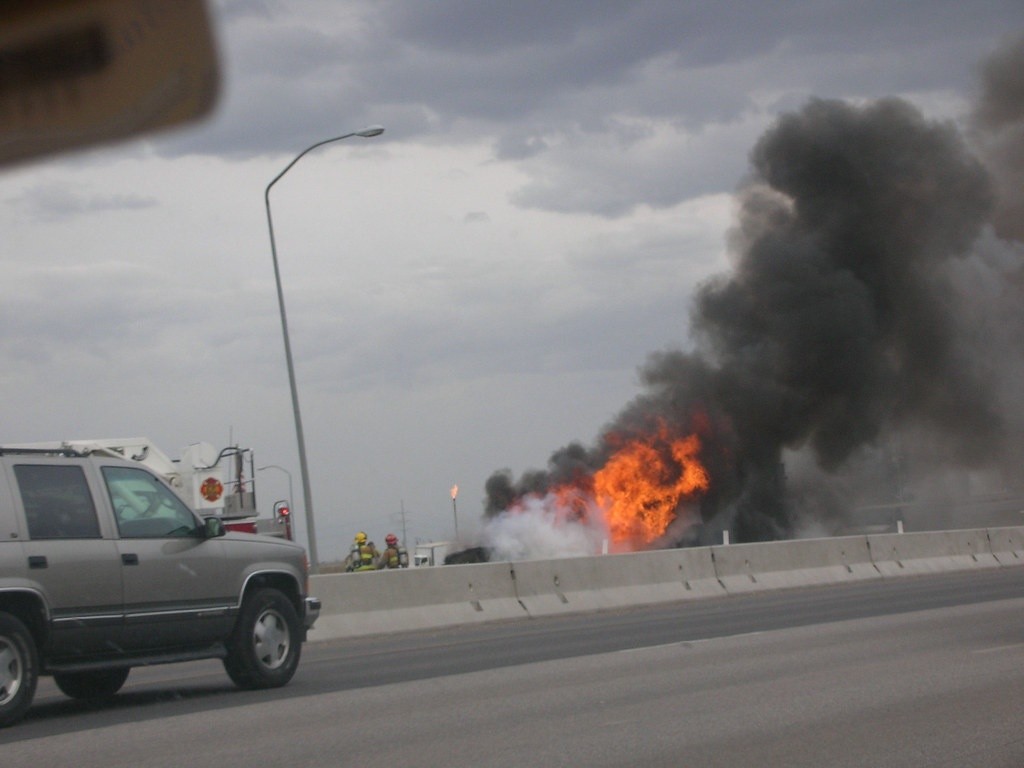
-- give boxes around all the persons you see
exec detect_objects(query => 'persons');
[343,532,382,571]
[377,534,401,570]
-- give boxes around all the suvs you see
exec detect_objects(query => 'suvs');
[0,444,323,729]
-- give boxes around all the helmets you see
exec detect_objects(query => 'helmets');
[386,535,396,544]
[355,532,367,542]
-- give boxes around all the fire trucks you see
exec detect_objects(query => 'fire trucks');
[61,439,296,554]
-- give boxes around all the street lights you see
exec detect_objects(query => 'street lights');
[265,128,384,576]
